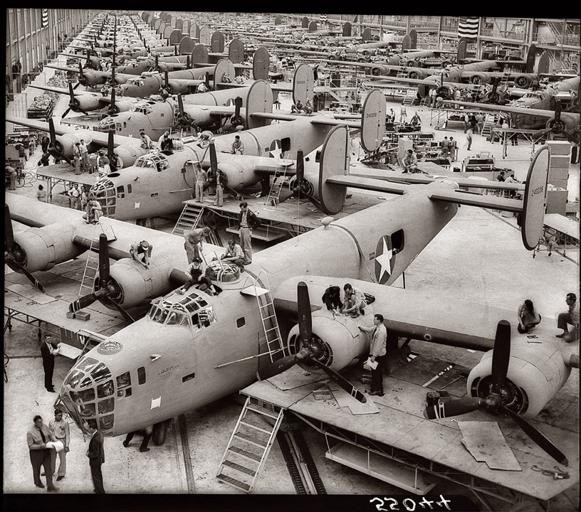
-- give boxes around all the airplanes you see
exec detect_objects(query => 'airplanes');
[2,124,581,467]
[5,5,579,220]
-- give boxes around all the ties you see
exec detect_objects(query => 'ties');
[39,429,46,443]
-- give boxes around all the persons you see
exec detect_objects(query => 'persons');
[2,9,581,493]
